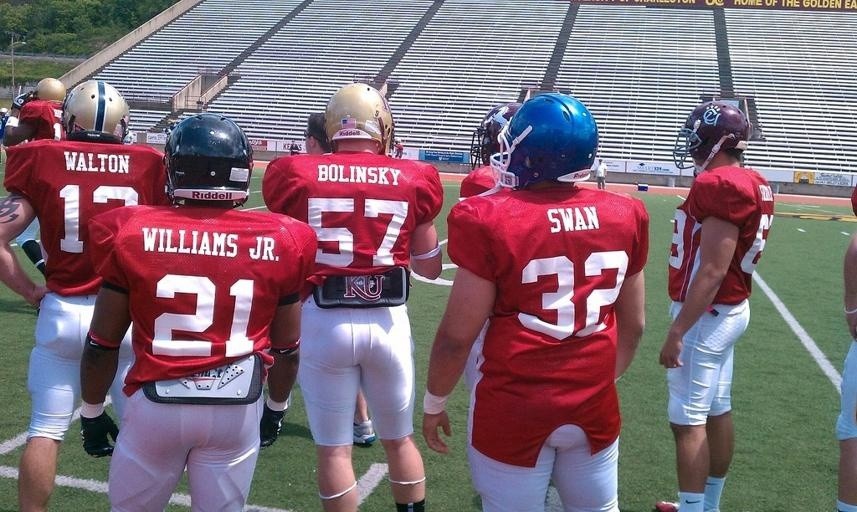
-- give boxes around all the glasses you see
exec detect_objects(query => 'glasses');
[304,130,320,142]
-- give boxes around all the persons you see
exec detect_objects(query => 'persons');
[303,111,331,155]
[289,138,300,155]
[80,113,318,511]
[0,107,9,162]
[656,101,774,512]
[352,388,377,446]
[458,102,537,391]
[0,80,173,511]
[261,83,444,511]
[4,77,67,145]
[834,182,857,512]
[393,142,403,160]
[422,92,650,512]
[594,159,607,189]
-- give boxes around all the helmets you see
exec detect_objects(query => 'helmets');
[37,78,65,101]
[324,83,395,157]
[60,80,130,143]
[490,93,598,191]
[471,102,523,170]
[673,101,749,178]
[162,113,253,201]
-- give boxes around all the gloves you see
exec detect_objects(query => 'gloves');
[80,399,119,458]
[260,396,288,450]
[11,91,33,110]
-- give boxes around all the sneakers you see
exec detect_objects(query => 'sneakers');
[353,417,377,444]
[656,501,680,512]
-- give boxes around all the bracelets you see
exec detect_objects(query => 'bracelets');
[266,394,289,411]
[80,398,105,419]
[842,304,855,317]
[423,387,451,416]
